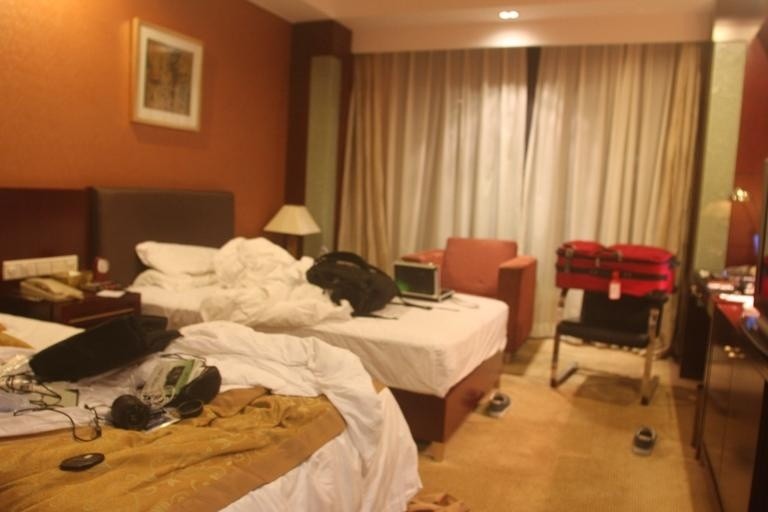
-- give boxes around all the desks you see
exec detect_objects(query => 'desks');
[679,268,754,448]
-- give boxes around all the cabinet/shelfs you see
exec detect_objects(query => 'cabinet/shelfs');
[694,303,767,511]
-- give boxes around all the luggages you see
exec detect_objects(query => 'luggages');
[556,241,679,304]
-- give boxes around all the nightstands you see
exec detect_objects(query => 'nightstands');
[1,287,142,328]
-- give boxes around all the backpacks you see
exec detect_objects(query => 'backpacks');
[307,252,430,318]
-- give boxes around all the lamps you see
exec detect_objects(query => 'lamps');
[262,203,322,259]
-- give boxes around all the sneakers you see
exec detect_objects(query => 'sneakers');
[488,392,511,418]
[632,426,655,455]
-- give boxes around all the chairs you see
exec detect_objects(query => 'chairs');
[402,234,537,363]
[548,287,663,405]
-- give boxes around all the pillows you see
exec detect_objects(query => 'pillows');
[132,240,220,294]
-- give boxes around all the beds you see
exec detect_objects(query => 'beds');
[1,312,422,512]
[87,183,511,462]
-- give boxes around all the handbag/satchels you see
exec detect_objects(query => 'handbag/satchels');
[31,314,184,384]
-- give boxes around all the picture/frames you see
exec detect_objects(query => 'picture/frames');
[129,16,206,134]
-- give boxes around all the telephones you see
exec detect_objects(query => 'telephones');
[20,277,84,302]
